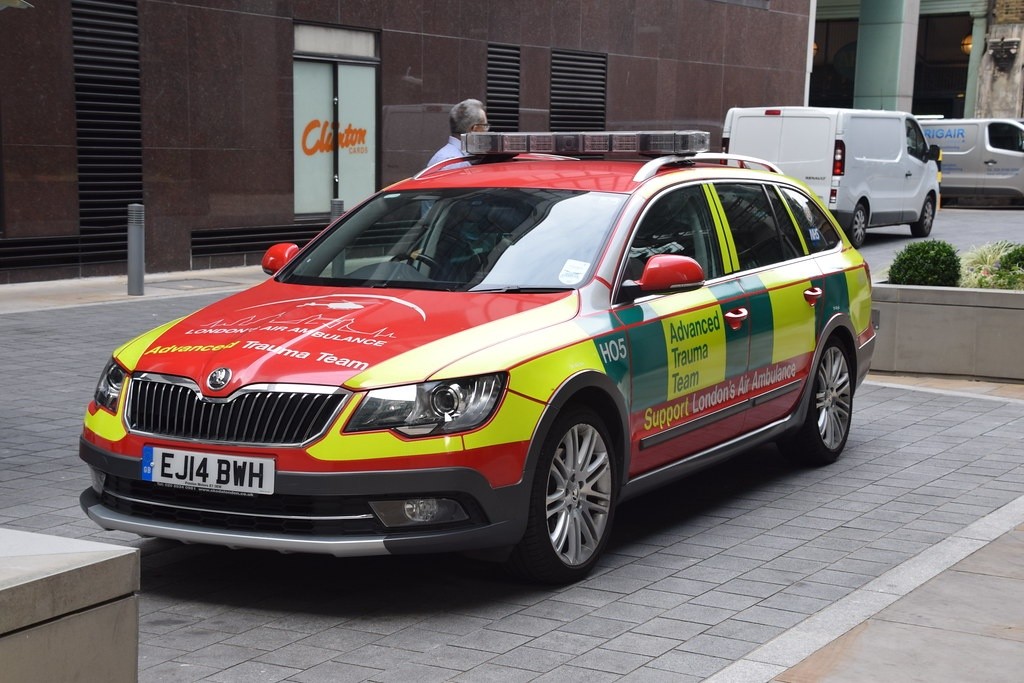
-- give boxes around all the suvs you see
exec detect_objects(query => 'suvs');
[79,150,878,586]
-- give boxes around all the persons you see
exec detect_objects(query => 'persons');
[421,99,487,216]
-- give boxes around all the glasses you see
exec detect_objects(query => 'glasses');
[472,124,491,132]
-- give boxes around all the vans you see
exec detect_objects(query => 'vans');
[915,116,1024,208]
[718,107,942,249]
